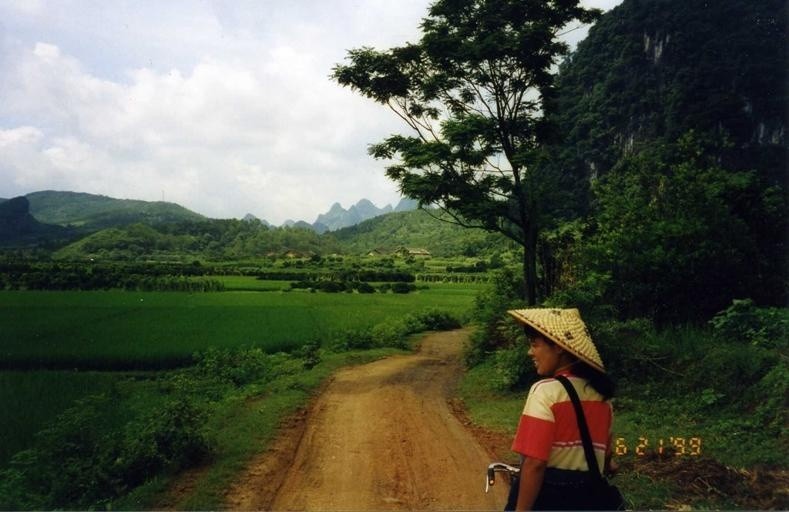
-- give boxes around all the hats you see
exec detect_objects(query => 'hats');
[508,308,605,377]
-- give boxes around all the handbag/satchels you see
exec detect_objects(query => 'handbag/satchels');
[506,466,625,511]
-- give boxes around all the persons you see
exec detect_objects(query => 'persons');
[506,306,621,511]
